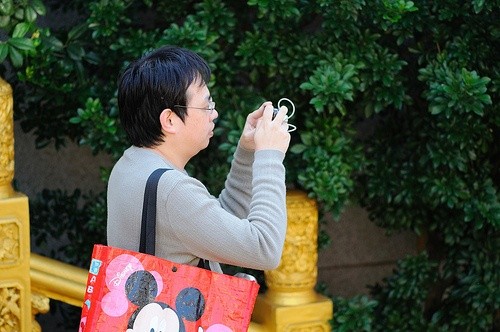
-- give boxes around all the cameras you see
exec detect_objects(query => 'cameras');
[271,109,288,124]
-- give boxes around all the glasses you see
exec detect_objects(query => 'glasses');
[174,96,216,114]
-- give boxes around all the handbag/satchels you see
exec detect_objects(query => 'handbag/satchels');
[78,167,258,332]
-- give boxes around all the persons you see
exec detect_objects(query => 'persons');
[107,45,290,274]
